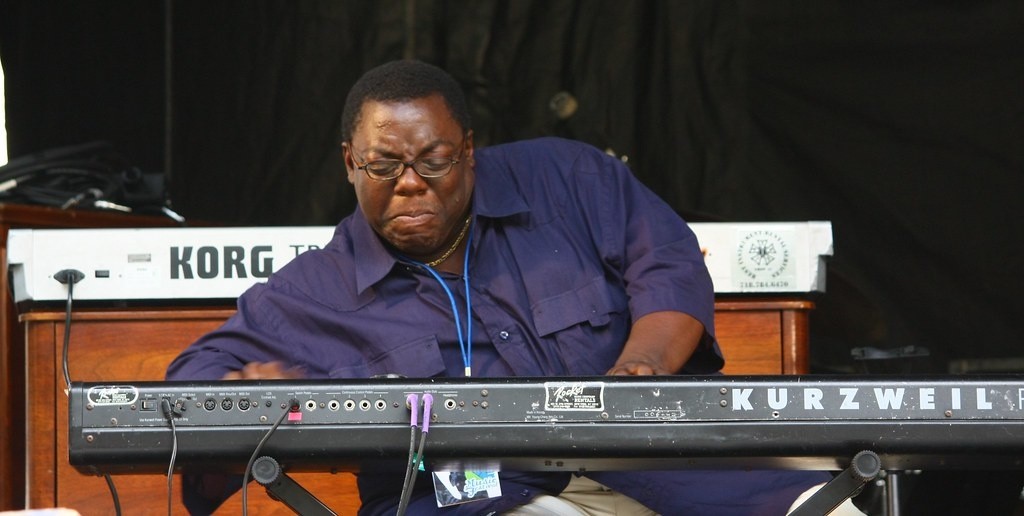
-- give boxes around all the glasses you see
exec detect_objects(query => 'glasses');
[348,135,466,182]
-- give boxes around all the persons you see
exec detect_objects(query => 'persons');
[162,57,874,516]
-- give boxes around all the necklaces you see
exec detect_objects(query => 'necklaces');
[423,212,473,268]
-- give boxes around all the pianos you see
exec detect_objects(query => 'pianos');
[65,375,1024,516]
